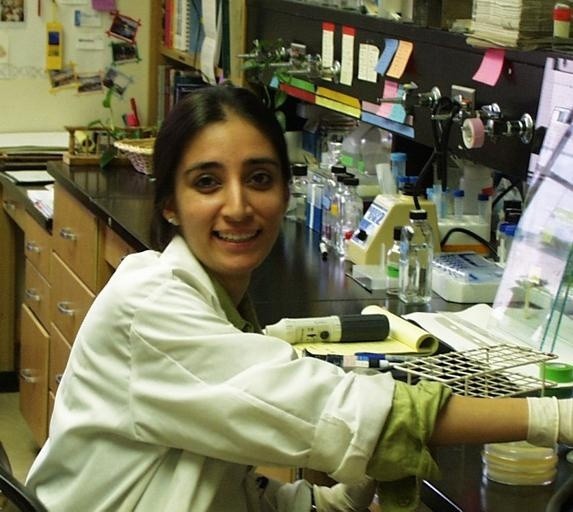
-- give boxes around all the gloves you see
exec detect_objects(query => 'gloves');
[312,475,377,512]
[525,396,572,448]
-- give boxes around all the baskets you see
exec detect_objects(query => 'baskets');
[113,137,157,175]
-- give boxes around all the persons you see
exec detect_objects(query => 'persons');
[18,85,572,510]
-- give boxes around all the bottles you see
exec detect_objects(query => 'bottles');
[285,152,517,305]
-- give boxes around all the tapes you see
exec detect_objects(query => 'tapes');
[462,116,487,150]
[539,363,573,383]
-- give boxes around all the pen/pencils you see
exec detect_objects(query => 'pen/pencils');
[319,240,328,257]
[314,354,421,369]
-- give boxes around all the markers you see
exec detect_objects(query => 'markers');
[264,314,389,343]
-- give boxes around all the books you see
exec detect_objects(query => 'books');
[462,0,572,54]
[155,0,230,129]
[261,302,441,367]
[0,130,96,221]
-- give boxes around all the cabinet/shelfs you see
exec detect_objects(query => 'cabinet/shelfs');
[0,0,573,512]
